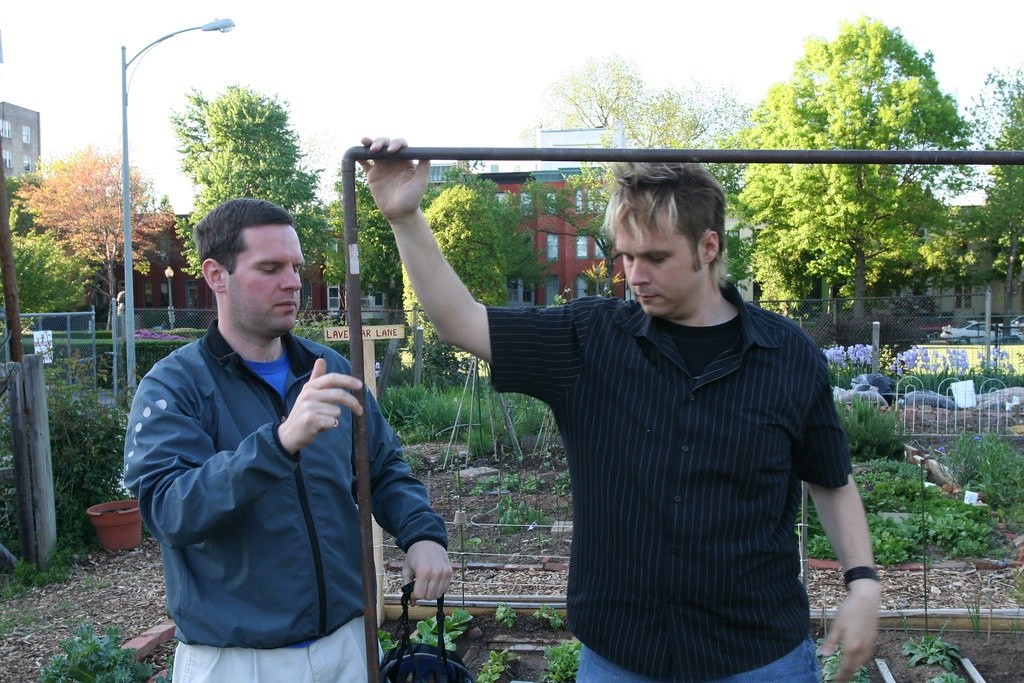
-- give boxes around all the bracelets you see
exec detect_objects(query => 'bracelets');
[843,566,880,589]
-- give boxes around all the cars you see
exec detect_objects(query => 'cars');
[939,319,1024,345]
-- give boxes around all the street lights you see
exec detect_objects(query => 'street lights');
[164,265,176,329]
[118,18,236,415]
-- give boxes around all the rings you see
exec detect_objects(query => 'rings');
[334,419,339,428]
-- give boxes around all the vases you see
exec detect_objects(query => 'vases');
[86,500,143,551]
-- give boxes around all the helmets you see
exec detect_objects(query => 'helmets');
[380,643,474,683]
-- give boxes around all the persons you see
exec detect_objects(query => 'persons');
[354,137,881,683]
[124,199,453,683]
